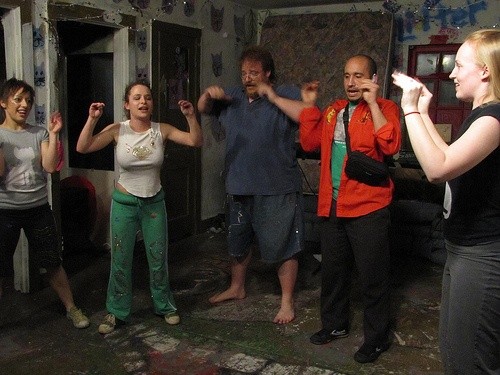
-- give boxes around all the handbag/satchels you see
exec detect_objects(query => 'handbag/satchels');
[342,103,389,188]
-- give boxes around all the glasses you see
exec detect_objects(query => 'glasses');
[240,69,264,80]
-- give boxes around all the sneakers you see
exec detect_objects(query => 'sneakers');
[355,340,392,363]
[98,314,118,333]
[66,306,89,328]
[310,327,349,345]
[164,311,180,324]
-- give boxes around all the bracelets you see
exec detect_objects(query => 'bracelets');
[402,111,420,117]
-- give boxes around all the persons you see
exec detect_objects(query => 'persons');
[298,55,401,364]
[76,82,203,334]
[197,49,303,323]
[391,29,500,375]
[0,78,89,329]
